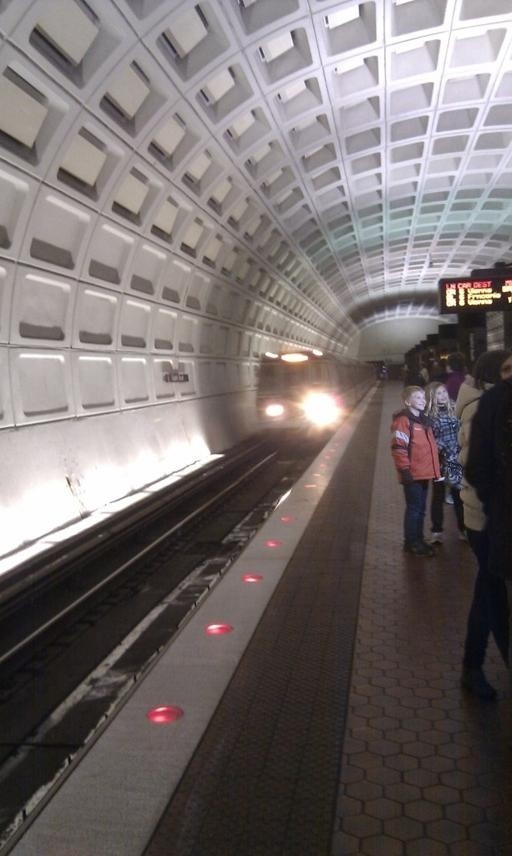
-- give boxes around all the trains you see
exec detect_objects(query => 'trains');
[252,342,384,457]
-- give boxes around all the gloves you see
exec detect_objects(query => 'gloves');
[401,468,414,484]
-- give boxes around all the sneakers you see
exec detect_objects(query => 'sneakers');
[459,529,469,541]
[404,542,429,554]
[461,664,498,697]
[431,531,444,544]
[445,493,454,504]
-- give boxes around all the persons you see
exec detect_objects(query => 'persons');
[465,374,512,673]
[389,347,512,559]
[453,347,512,709]
